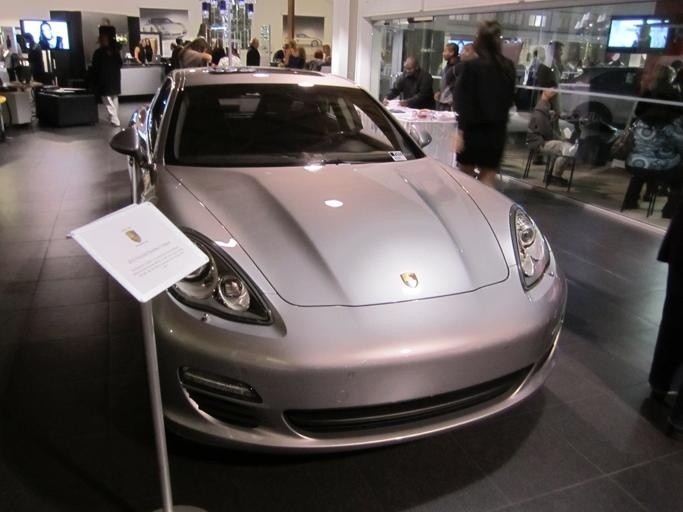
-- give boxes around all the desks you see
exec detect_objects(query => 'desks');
[353,104,459,169]
[34,90,98,129]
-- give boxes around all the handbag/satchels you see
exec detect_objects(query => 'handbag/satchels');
[611,128,634,161]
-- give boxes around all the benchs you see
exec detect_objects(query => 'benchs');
[521,148,576,194]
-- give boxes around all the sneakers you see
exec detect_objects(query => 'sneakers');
[548,173,568,186]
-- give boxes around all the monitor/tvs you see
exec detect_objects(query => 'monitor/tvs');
[20,19,71,53]
[606,15,672,53]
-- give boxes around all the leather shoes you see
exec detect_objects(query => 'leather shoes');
[640,391,682,439]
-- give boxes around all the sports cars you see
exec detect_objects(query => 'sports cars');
[106,64,573,456]
[142,17,187,41]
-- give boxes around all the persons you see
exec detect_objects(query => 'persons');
[384,56,436,110]
[92,34,121,125]
[525,47,559,109]
[433,42,477,111]
[620,60,682,218]
[632,24,651,46]
[133,39,330,75]
[453,20,514,190]
[641,160,681,433]
[35,21,63,49]
[527,83,575,187]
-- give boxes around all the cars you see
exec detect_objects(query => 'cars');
[507,60,682,136]
[285,33,322,48]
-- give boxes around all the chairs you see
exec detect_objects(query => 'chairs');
[0,96,12,143]
